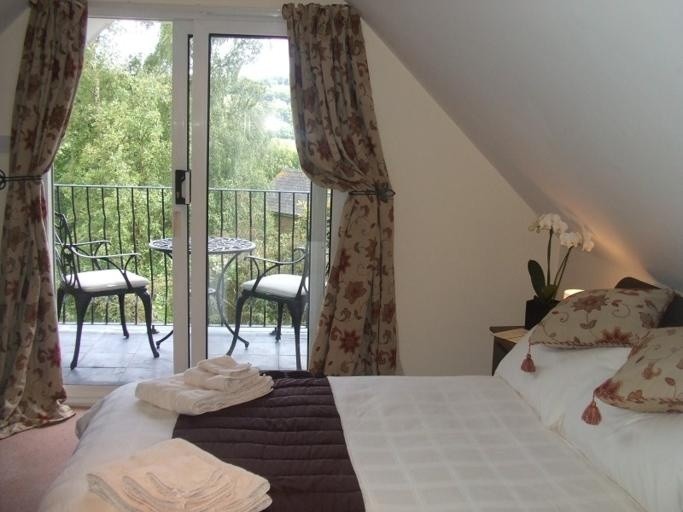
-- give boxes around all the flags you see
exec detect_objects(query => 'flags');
[523,298,559,330]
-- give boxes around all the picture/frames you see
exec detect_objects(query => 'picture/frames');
[144,236,257,351]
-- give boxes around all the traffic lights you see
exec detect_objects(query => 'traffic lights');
[521,284,676,376]
[582,325,681,432]
[487,326,633,429]
[551,378,683,512]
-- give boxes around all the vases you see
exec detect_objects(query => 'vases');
[524,211,594,302]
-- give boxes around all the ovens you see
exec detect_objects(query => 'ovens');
[33,375,647,511]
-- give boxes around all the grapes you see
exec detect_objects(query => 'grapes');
[488,327,532,374]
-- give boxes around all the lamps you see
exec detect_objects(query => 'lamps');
[226,218,330,370]
[53,210,162,370]
